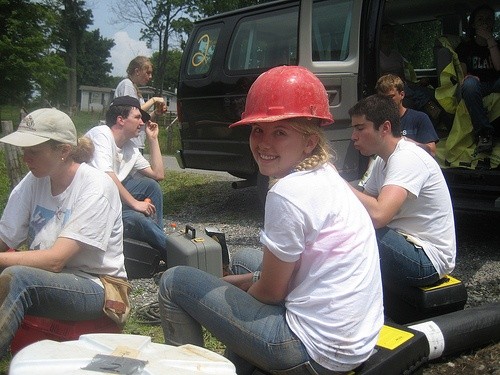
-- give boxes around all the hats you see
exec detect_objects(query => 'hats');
[108,95,151,123]
[0,108,77,148]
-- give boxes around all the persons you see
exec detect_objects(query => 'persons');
[158,64,384,375]
[112,56,168,154]
[451,6,500,156]
[375,72,442,163]
[81,96,168,265]
[0,107,132,362]
[345,93,456,286]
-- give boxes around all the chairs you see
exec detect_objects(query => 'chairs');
[434,34,500,114]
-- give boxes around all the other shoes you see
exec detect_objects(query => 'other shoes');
[475,130,494,153]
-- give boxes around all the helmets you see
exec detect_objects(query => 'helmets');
[227,65,334,129]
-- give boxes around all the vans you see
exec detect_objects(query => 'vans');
[173,0,500,224]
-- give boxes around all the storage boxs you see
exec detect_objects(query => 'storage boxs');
[337,320,431,375]
[385,274,468,326]
[165,224,224,277]
[9,315,120,353]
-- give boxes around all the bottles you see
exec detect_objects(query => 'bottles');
[169,223,177,235]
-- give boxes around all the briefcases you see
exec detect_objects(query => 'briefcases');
[165,224,223,277]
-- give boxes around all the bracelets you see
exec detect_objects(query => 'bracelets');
[487,44,498,49]
[253,270,260,284]
[151,96,156,105]
[485,36,494,40]
[153,110,159,117]
